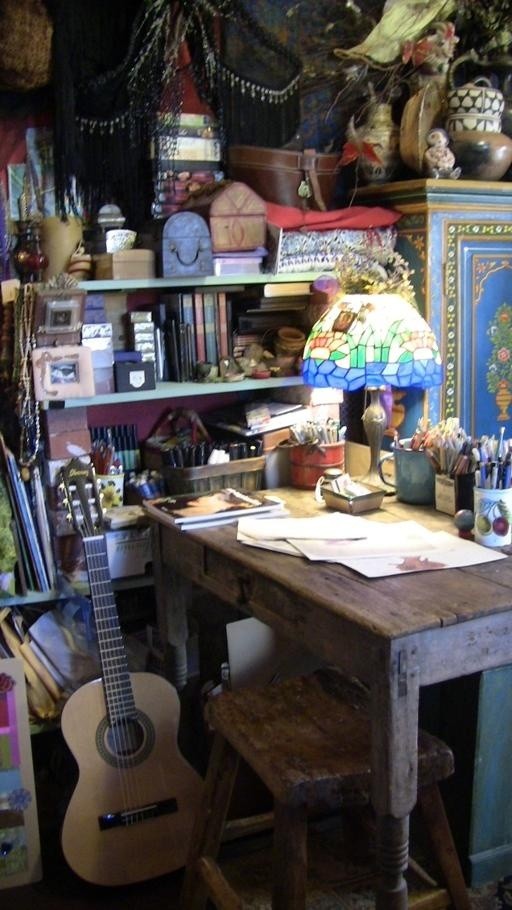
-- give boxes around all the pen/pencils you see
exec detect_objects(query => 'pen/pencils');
[393,416,460,450]
[475,426,512,489]
[289,418,347,444]
[428,430,487,479]
[92,439,120,475]
[163,440,263,467]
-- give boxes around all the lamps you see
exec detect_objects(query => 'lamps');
[303,293,441,497]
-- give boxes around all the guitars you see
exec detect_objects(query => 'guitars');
[62,456,205,884]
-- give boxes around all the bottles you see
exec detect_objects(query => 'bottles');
[275,326,306,358]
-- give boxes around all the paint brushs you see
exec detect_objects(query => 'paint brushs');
[129,468,164,501]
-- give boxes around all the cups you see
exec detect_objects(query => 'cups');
[378,437,435,505]
[473,486,512,547]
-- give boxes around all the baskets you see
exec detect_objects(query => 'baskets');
[320,478,386,513]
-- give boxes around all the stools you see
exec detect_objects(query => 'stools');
[183,674,473,909]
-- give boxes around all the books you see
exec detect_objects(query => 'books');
[233,281,314,359]
[159,293,233,382]
[142,486,289,531]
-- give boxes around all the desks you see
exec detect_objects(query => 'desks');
[142,488,510,907]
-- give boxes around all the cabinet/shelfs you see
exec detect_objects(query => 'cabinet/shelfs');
[0,269,341,738]
